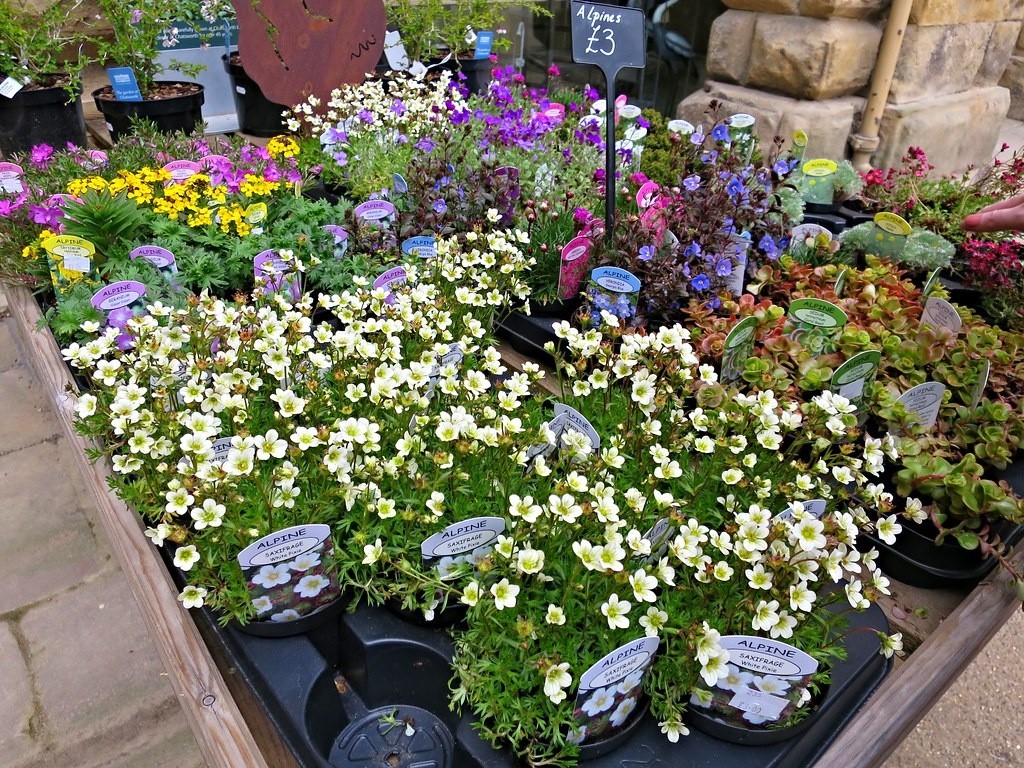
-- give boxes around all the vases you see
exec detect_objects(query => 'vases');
[429,43,501,89]
[1,71,86,156]
[94,79,205,139]
[221,50,309,135]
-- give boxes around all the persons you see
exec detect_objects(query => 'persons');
[959,192,1024,233]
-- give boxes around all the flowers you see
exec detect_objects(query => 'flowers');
[2,1,1024,767]
